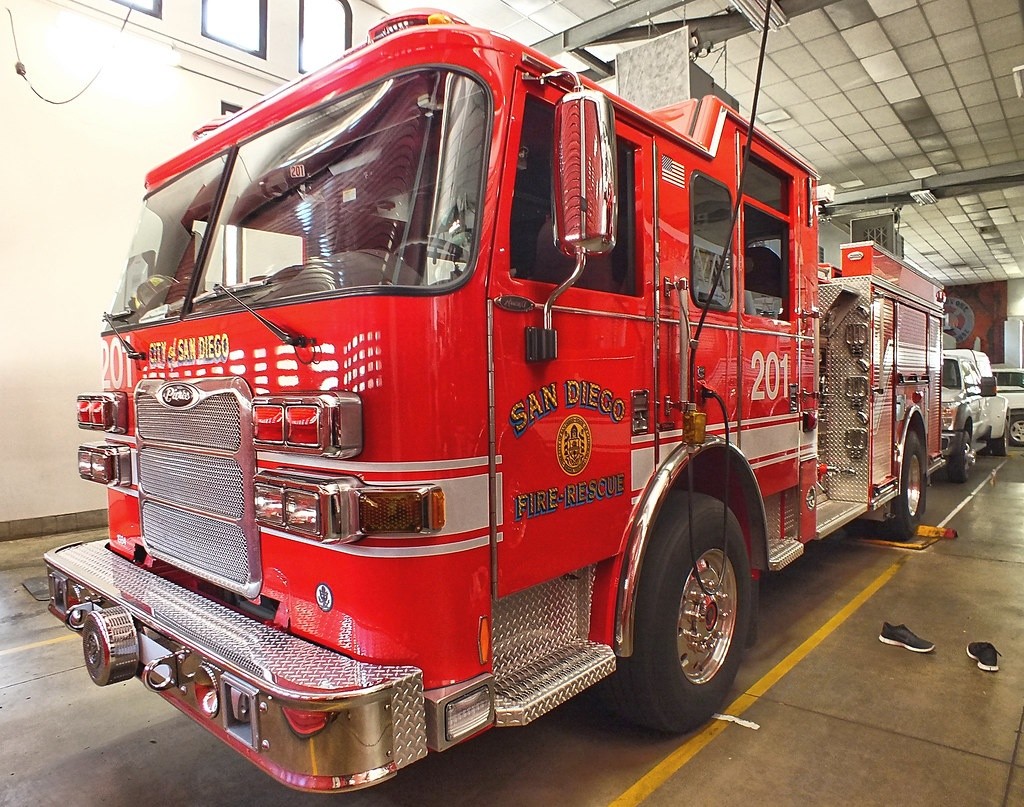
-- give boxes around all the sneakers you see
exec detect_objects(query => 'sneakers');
[966,642,1004,671]
[878,622,936,652]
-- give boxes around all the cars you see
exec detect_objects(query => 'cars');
[993,368,1024,448]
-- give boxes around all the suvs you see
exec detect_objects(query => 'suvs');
[942,349,1013,484]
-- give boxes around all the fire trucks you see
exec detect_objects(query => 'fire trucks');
[22,5,948,796]
